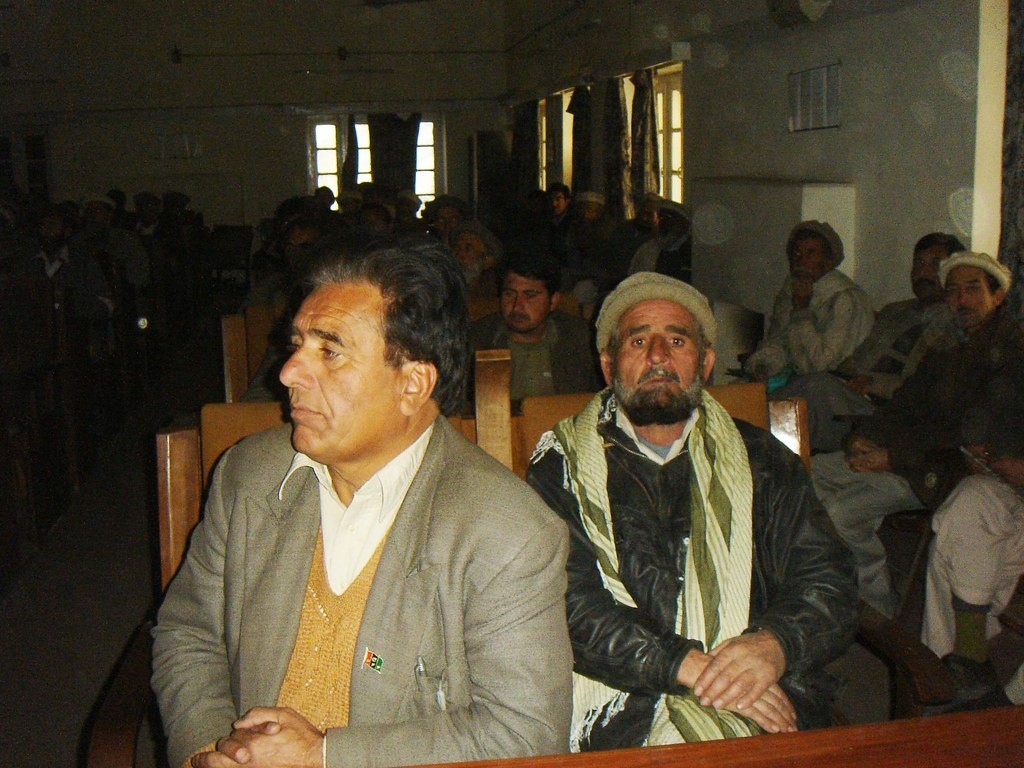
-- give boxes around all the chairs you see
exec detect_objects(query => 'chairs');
[78,312,812,767]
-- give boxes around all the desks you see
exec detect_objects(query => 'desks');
[401,700,1024,766]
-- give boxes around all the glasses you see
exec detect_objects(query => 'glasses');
[910,256,940,271]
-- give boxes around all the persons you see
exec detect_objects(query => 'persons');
[0,190,201,406]
[901,352,1024,717]
[247,178,697,303]
[459,254,609,416]
[802,250,1009,630]
[520,269,861,753]
[767,231,966,452]
[740,218,871,388]
[147,229,575,768]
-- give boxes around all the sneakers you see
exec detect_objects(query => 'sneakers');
[909,653,998,712]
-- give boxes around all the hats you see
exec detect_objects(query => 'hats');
[84,192,117,210]
[594,271,717,358]
[786,220,844,268]
[397,189,422,209]
[425,194,475,220]
[450,220,508,267]
[939,252,1012,293]
[574,190,606,207]
[658,199,690,222]
[634,191,662,204]
[337,190,364,205]
